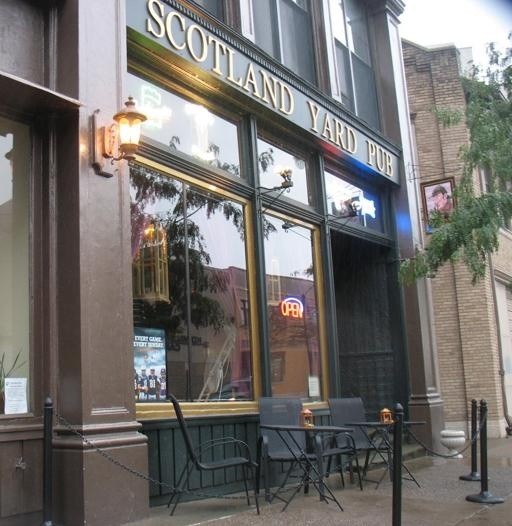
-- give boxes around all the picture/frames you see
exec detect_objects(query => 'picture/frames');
[419,175,459,233]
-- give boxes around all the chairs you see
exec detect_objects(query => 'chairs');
[163,390,263,517]
[326,398,394,488]
[256,394,362,494]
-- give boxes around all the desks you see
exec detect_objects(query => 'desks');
[343,421,428,489]
[256,422,357,512]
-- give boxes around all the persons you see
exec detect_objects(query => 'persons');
[429,186,451,212]
[132,365,165,402]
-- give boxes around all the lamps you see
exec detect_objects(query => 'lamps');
[325,197,363,239]
[257,167,293,214]
[90,96,147,178]
